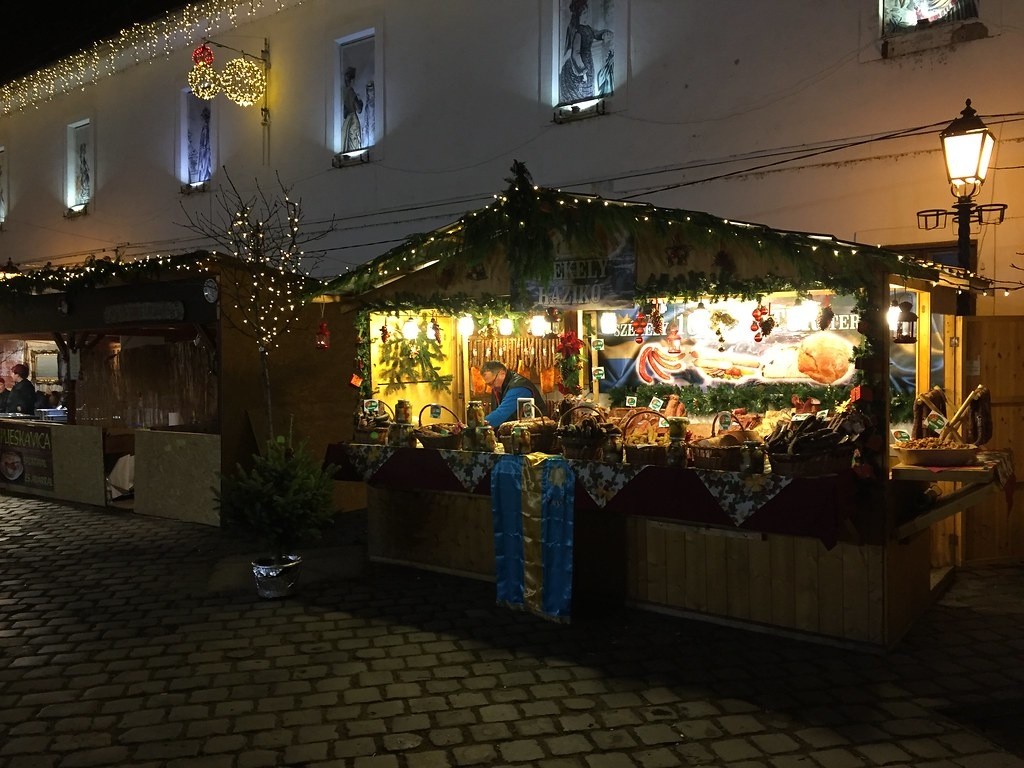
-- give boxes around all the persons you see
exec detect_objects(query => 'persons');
[5,364,35,415]
[0,378,10,413]
[480,361,547,438]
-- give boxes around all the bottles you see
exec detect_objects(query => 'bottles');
[603,434,623,461]
[510,427,531,454]
[668,417,689,438]
[477,427,495,452]
[400,424,416,447]
[668,438,688,468]
[740,441,764,474]
[395,400,411,424]
[462,427,476,450]
[388,423,400,446]
[467,401,484,427]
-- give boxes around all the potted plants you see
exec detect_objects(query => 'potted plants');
[209,412,341,600]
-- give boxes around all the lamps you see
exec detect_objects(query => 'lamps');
[426,310,440,339]
[402,310,419,340]
[687,297,711,336]
[187,36,271,127]
[893,286,917,343]
[887,288,902,331]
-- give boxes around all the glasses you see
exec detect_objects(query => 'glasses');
[487,370,501,387]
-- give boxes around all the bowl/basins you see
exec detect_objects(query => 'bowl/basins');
[889,442,979,466]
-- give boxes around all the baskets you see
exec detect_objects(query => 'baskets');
[769,441,858,478]
[689,413,766,472]
[553,405,610,460]
[499,402,557,453]
[414,405,464,450]
[354,400,394,444]
[889,444,979,466]
[623,411,673,464]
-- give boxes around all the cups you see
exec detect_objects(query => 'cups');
[167,412,180,426]
